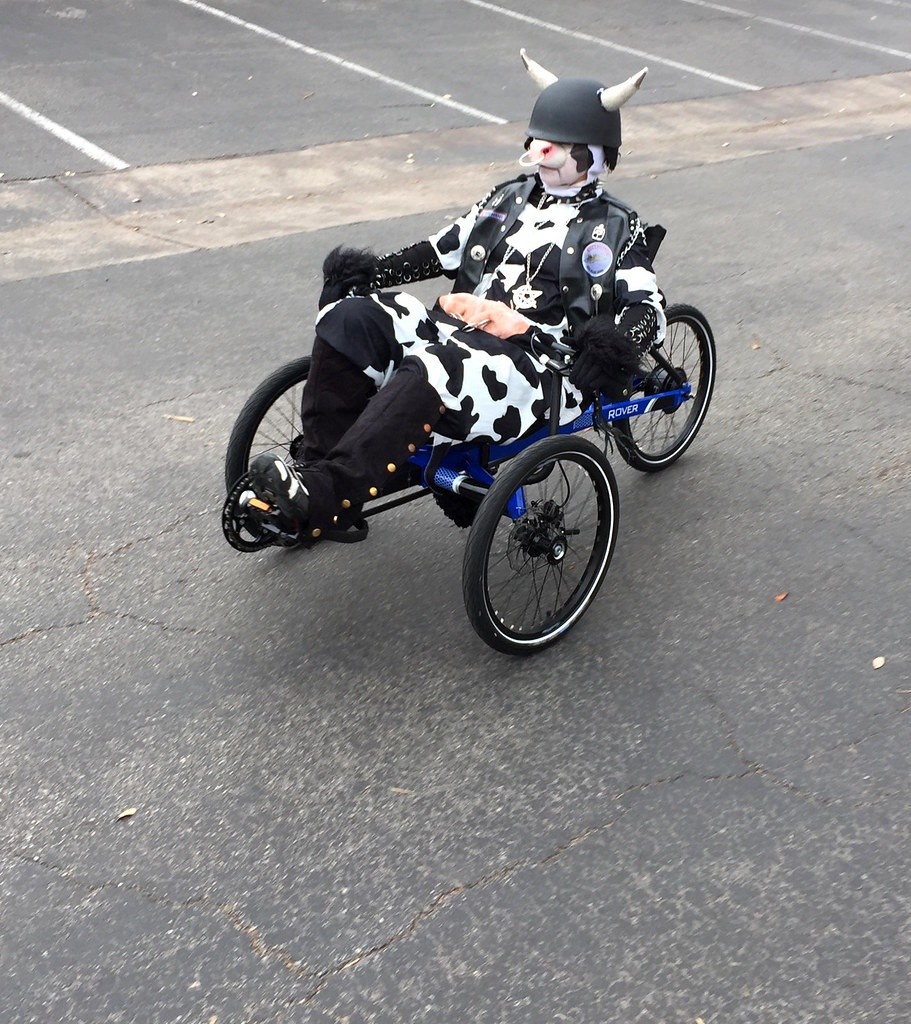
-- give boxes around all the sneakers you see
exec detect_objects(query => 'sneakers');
[250,453,312,535]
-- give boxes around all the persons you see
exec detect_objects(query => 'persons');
[250,48,666,549]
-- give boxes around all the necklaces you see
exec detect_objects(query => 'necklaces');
[495,188,592,311]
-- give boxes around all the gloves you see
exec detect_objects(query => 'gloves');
[317,271,365,310]
[560,333,622,395]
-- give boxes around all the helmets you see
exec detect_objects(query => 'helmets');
[518,45,649,146]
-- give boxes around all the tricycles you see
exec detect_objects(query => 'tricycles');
[223,224,716,654]
